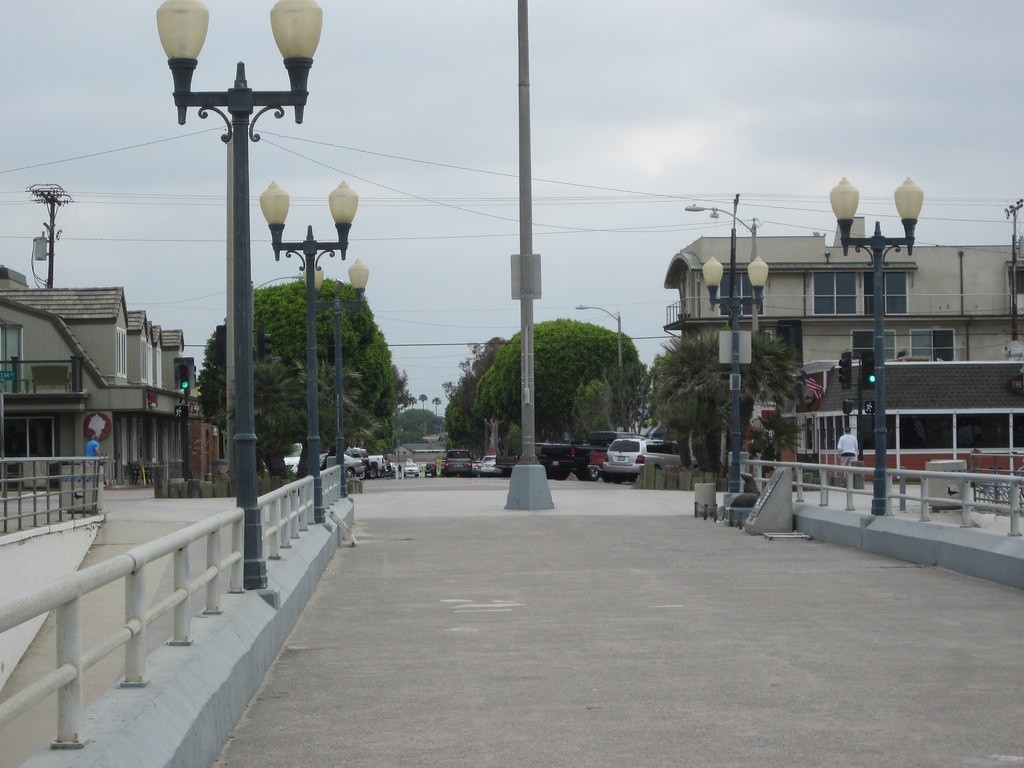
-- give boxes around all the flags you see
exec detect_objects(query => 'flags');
[799,369,823,399]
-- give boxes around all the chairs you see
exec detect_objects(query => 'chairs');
[127,459,154,485]
[29,365,73,394]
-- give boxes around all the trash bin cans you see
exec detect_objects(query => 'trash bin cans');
[59,462,107,513]
[212,460,228,482]
[22,461,47,489]
[925,459,968,510]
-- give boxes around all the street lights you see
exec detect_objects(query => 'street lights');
[303,259,369,495]
[829,176,925,516]
[704,256,769,494]
[258,179,358,522]
[684,205,759,330]
[157,0,323,591]
[576,306,622,364]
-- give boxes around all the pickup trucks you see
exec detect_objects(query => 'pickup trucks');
[535,431,645,481]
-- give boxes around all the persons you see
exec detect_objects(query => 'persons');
[837,427,858,479]
[86,435,101,457]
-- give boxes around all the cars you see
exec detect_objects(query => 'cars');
[404,463,419,477]
[263,443,302,474]
[473,455,517,477]
[327,452,364,478]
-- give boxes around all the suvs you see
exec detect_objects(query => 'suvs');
[425,464,436,476]
[445,449,473,477]
[603,436,698,482]
[344,447,392,479]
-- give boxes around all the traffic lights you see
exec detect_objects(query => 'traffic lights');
[861,351,875,388]
[175,406,189,419]
[843,400,853,414]
[172,357,195,390]
[839,351,852,390]
[865,401,875,413]
